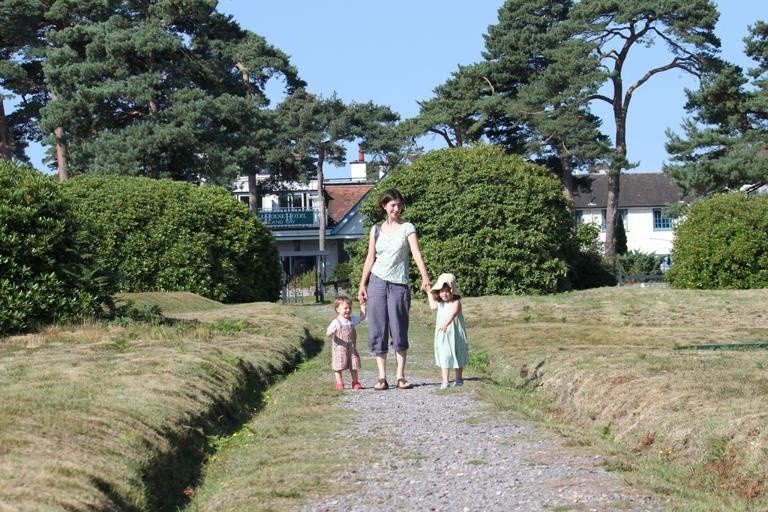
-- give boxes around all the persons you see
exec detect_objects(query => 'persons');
[358,186,431,391]
[423,273,470,391]
[325,296,368,390]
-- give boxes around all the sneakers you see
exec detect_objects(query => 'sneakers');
[441,383,450,389]
[352,381,362,390]
[336,383,344,389]
[454,380,464,387]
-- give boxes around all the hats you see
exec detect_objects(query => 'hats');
[431,273,463,299]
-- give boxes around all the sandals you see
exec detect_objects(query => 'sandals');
[397,378,413,388]
[374,378,388,390]
